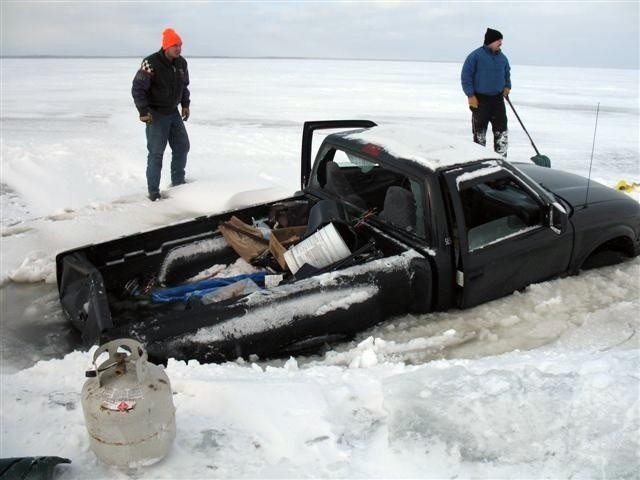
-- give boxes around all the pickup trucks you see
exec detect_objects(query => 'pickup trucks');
[56,119,640,370]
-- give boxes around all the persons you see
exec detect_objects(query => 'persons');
[460,28,511,158]
[132,28,191,202]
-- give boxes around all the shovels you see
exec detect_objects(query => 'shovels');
[504,93,552,169]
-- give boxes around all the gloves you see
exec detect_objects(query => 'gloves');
[139,111,153,125]
[181,105,190,122]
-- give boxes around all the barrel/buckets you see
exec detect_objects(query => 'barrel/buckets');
[302,199,357,253]
[283,222,351,276]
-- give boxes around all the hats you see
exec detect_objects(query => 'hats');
[483,27,503,46]
[161,28,182,50]
